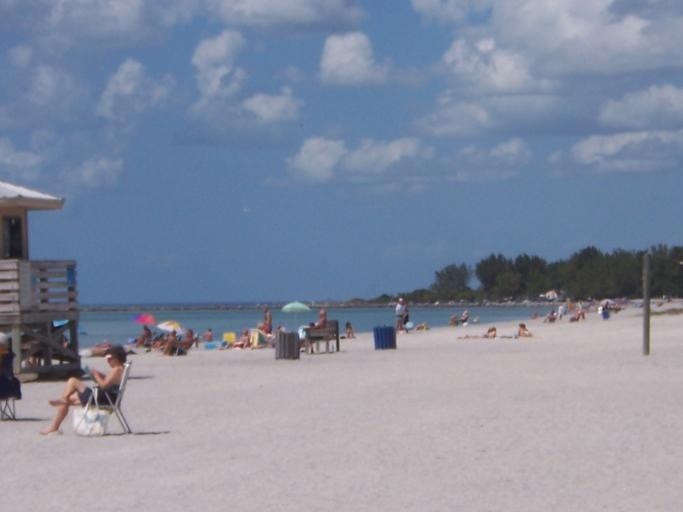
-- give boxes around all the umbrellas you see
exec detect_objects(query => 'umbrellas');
[127,313,155,328]
[281,301,309,320]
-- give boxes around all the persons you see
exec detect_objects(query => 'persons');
[261,303,272,333]
[632,296,672,309]
[448,309,480,328]
[394,298,411,333]
[18,333,69,369]
[315,309,328,326]
[457,327,496,340]
[79,321,355,360]
[0,333,15,384]
[38,344,128,436]
[517,323,531,337]
[531,297,627,324]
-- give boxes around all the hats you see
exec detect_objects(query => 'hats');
[398,298,402,301]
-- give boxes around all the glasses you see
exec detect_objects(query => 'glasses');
[104,351,122,360]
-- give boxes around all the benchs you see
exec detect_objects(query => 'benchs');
[303,319,340,355]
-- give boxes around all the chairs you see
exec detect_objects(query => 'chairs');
[0,337,22,421]
[73,362,134,433]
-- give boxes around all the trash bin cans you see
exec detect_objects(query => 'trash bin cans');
[374,326,396,349]
[275,331,300,360]
